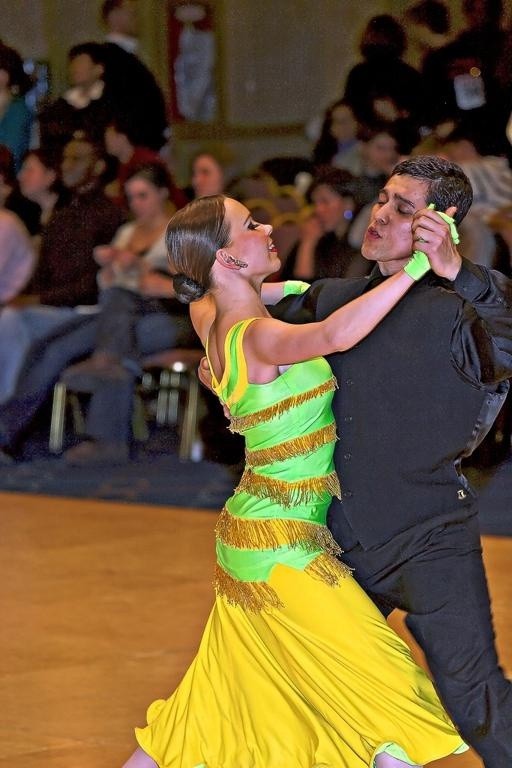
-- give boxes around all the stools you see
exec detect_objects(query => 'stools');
[50,346,211,455]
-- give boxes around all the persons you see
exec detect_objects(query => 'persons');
[0,135,124,452]
[17,150,68,238]
[410,97,512,265]
[344,12,432,157]
[60,146,243,468]
[105,107,187,217]
[0,169,39,307]
[197,162,512,768]
[419,0,511,161]
[1,161,179,468]
[190,151,225,199]
[122,197,470,768]
[191,166,369,486]
[41,43,125,188]
[101,0,173,151]
[0,39,38,174]
[352,116,424,208]
[313,94,383,177]
[0,143,44,237]
[289,168,377,280]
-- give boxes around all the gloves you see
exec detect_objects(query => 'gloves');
[284,280,312,298]
[403,203,460,282]
